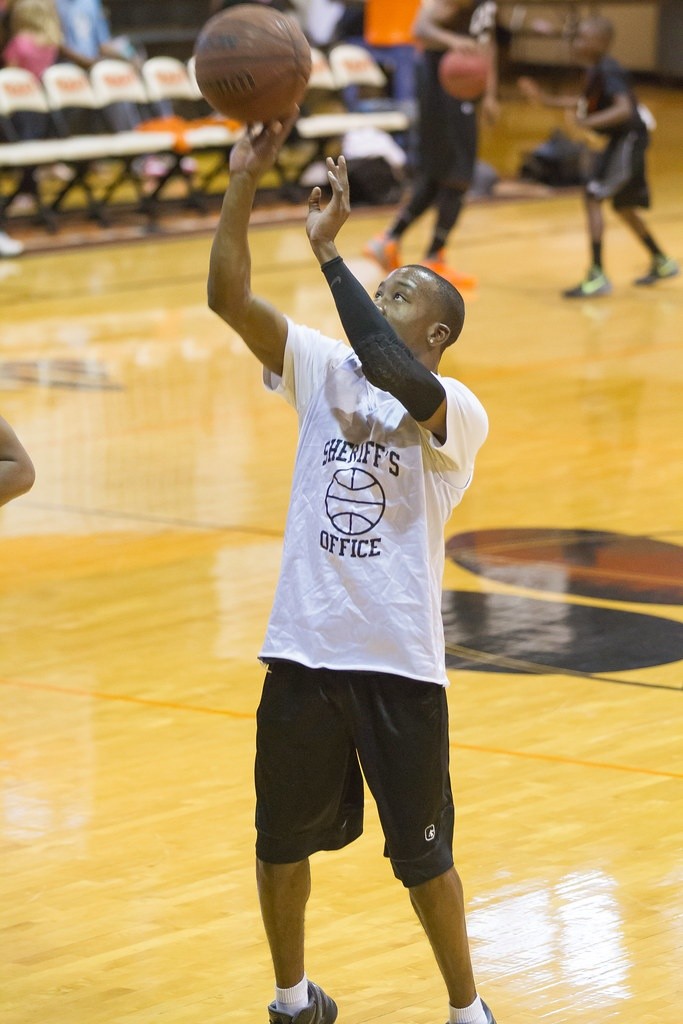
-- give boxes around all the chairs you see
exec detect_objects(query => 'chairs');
[0,36,411,233]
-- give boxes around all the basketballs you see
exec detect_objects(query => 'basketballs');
[194,4,311,120]
[437,50,489,98]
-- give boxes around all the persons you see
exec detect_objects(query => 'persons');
[206,102,499,1024]
[516,13,679,301]
[0,0,201,258]
[0,413,35,509]
[359,0,499,293]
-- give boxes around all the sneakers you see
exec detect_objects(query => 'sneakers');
[446,996,498,1024]
[268,979,338,1024]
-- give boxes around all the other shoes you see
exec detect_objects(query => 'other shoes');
[365,237,402,272]
[564,272,610,296]
[421,257,475,290]
[636,258,678,285]
